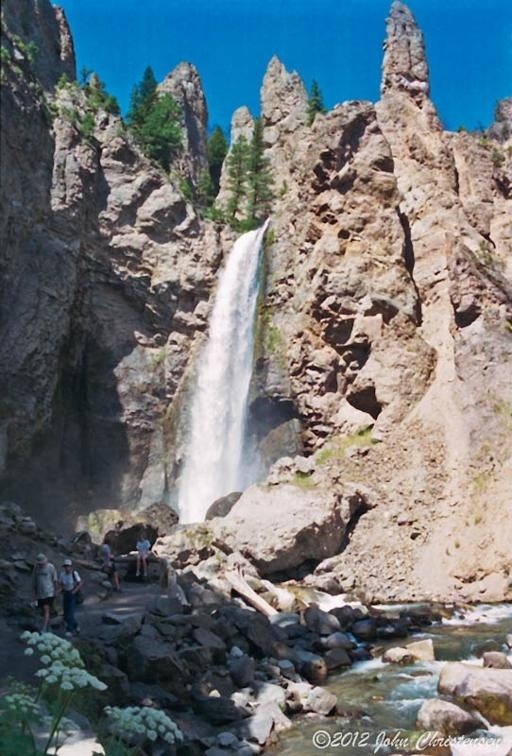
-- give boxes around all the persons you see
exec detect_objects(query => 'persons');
[99,538,124,593]
[134,530,152,579]
[56,558,85,640]
[33,552,60,634]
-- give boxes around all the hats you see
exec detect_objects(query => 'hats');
[60,559,73,567]
[34,554,47,565]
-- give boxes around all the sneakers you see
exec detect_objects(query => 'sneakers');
[65,624,81,638]
[38,629,49,635]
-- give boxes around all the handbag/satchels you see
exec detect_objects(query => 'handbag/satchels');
[74,587,84,605]
